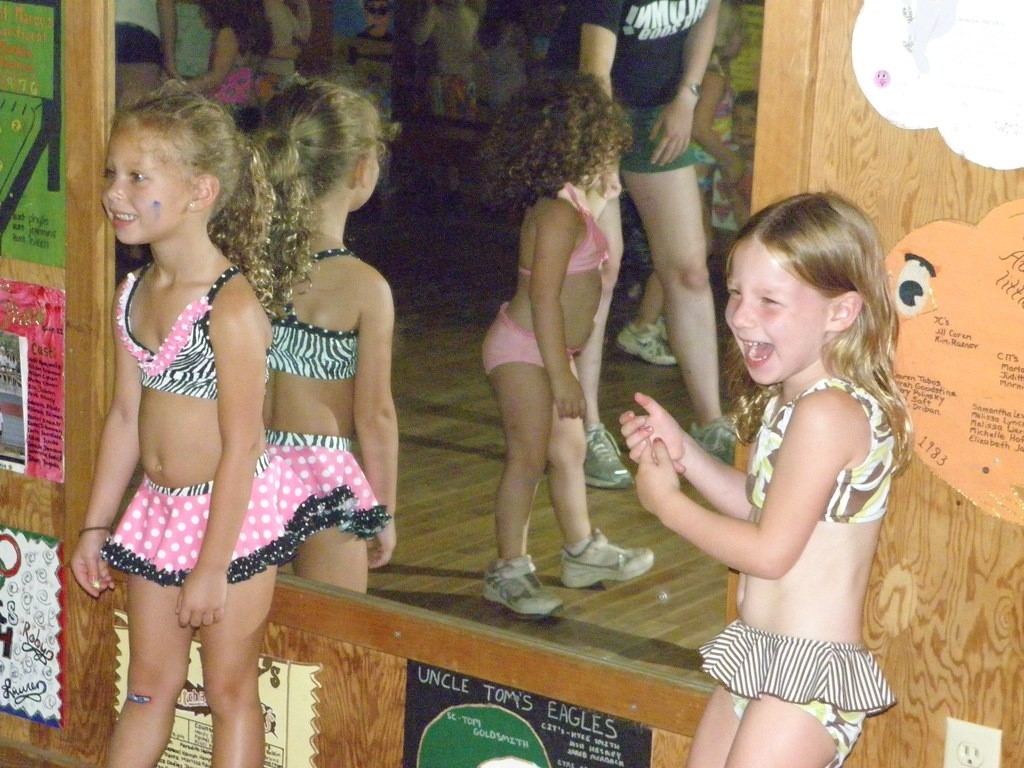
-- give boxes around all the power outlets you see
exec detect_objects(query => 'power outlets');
[944,715,1004,768]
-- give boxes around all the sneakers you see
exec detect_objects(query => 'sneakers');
[560,527,656,588]
[690,417,740,465]
[581,423,635,489]
[615,316,675,365]
[480,552,564,619]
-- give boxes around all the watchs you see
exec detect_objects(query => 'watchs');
[675,81,704,96]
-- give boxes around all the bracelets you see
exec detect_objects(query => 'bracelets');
[79,527,113,537]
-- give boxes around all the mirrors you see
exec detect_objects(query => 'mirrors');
[112,0,771,692]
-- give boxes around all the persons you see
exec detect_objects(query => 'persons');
[69,94,292,767]
[619,193,914,768]
[255,81,396,595]
[115,1,563,272]
[481,75,652,618]
[616,0,744,364]
[543,0,736,490]
